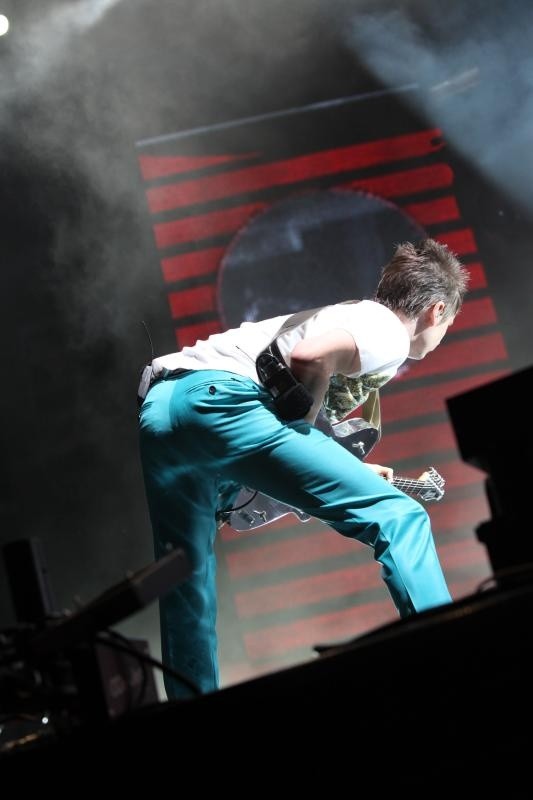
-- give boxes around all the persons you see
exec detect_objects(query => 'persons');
[136,239,470,704]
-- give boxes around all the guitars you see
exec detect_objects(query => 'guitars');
[221,412,447,532]
